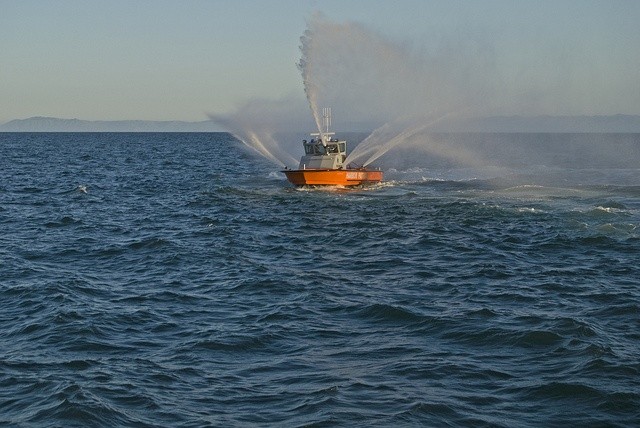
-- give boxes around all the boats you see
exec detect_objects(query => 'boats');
[280,108,384,186]
